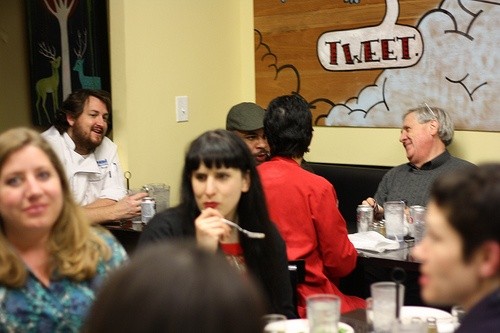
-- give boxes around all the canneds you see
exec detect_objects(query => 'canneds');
[409,205,425,238]
[356,205,374,232]
[141,197,156,225]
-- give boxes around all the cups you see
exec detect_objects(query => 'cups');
[370,282,404,333]
[412,207,426,242]
[383,201,405,242]
[306,294,341,333]
[155,185,171,213]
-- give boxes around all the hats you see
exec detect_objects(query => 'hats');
[226,102,265,131]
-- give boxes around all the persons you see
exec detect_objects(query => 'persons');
[253,93,371,318]
[227,102,272,170]
[38,87,151,250]
[79,241,266,333]
[0,126,128,333]
[408,161,500,333]
[339,105,481,306]
[134,128,302,318]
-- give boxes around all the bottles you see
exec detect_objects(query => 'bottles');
[409,317,423,333]
[426,317,438,333]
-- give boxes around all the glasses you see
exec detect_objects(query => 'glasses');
[417,100,437,118]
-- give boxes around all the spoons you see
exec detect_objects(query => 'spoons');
[221,218,264,238]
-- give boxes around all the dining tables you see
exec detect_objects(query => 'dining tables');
[335,224,454,314]
[97,209,160,260]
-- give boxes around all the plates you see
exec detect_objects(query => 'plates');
[398,306,456,333]
[263,318,355,333]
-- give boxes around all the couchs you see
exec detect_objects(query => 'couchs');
[300,160,453,224]
